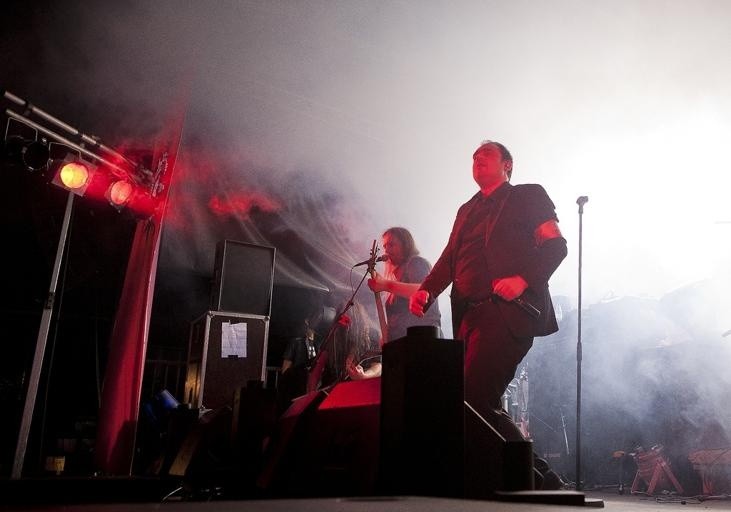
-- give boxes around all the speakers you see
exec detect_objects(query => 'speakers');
[376,335,468,497]
[210,237,277,317]
[313,375,509,496]
[266,388,329,496]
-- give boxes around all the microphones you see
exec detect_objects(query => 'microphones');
[514,296,541,321]
[354,255,389,268]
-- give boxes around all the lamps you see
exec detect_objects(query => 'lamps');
[19,131,140,213]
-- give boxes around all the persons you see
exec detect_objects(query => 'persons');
[366,225,447,353]
[279,309,326,400]
[405,138,570,490]
[306,299,384,397]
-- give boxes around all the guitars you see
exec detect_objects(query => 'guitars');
[367,240,390,344]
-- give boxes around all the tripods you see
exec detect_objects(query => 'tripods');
[578,350,657,495]
